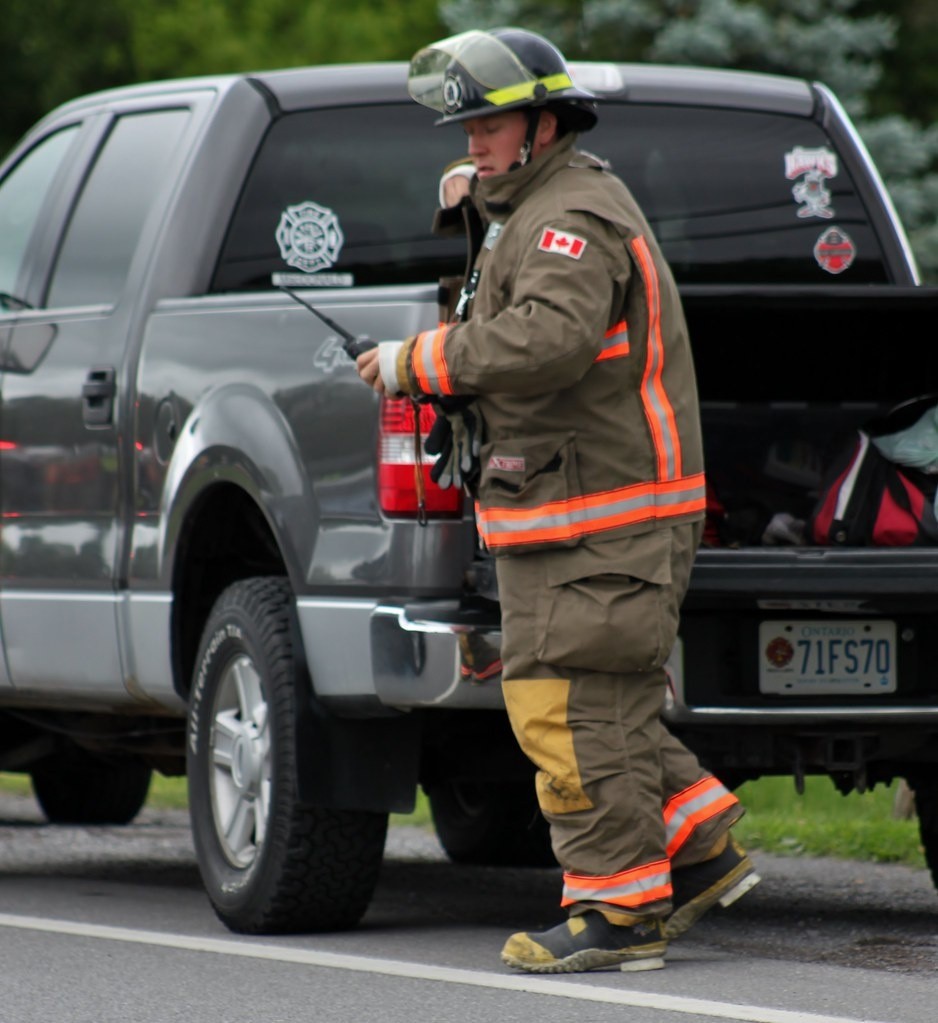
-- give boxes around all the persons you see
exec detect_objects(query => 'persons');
[356,28,760,973]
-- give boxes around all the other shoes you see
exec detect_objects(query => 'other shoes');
[657,829,762,941]
[501,910,667,972]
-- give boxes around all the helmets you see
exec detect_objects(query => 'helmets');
[408,28,606,136]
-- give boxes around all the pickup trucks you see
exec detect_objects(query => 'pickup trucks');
[0,58,938,935]
